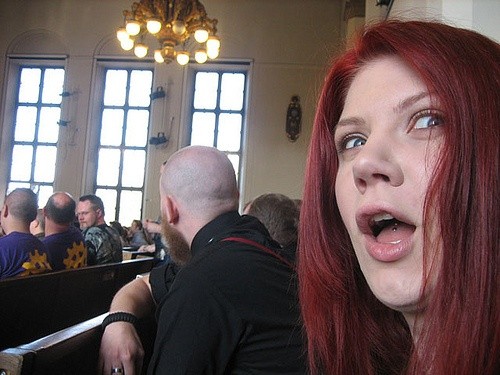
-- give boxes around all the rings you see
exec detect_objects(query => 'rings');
[111,368,124,375]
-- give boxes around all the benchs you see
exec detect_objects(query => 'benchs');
[0,246,157,375]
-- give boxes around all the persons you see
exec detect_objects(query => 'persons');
[28,208,45,239]
[295,15,500,375]
[110,208,164,256]
[143,158,171,261]
[0,187,53,280]
[36,191,89,274]
[76,192,123,268]
[97,146,309,375]
[242,191,308,250]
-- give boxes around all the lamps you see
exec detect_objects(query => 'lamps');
[116,0,221,67]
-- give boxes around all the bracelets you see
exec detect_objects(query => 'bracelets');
[101,312,140,332]
